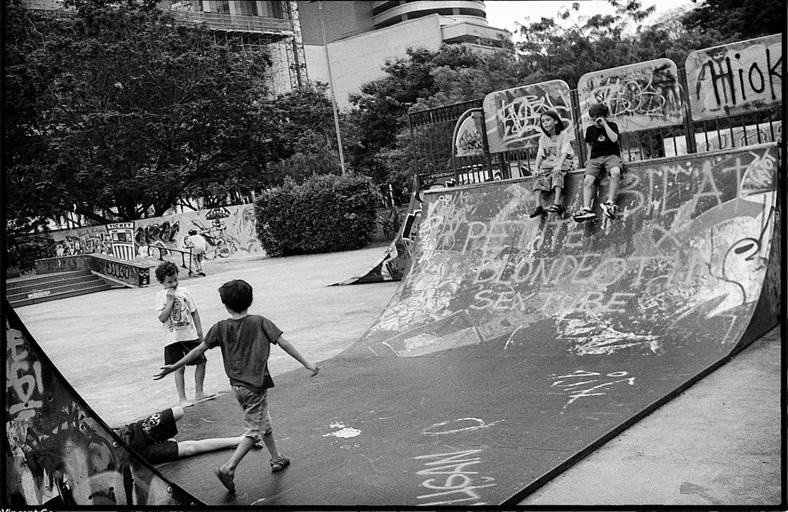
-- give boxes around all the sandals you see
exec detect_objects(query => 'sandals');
[529,205,544,218]
[270,457,290,473]
[214,464,236,494]
[548,202,562,212]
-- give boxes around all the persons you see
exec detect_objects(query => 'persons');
[111,406,262,465]
[185,228,207,273]
[153,279,321,493]
[571,104,625,223]
[154,260,217,409]
[527,110,576,219]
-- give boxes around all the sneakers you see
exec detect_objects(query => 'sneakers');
[599,200,618,219]
[573,207,596,221]
